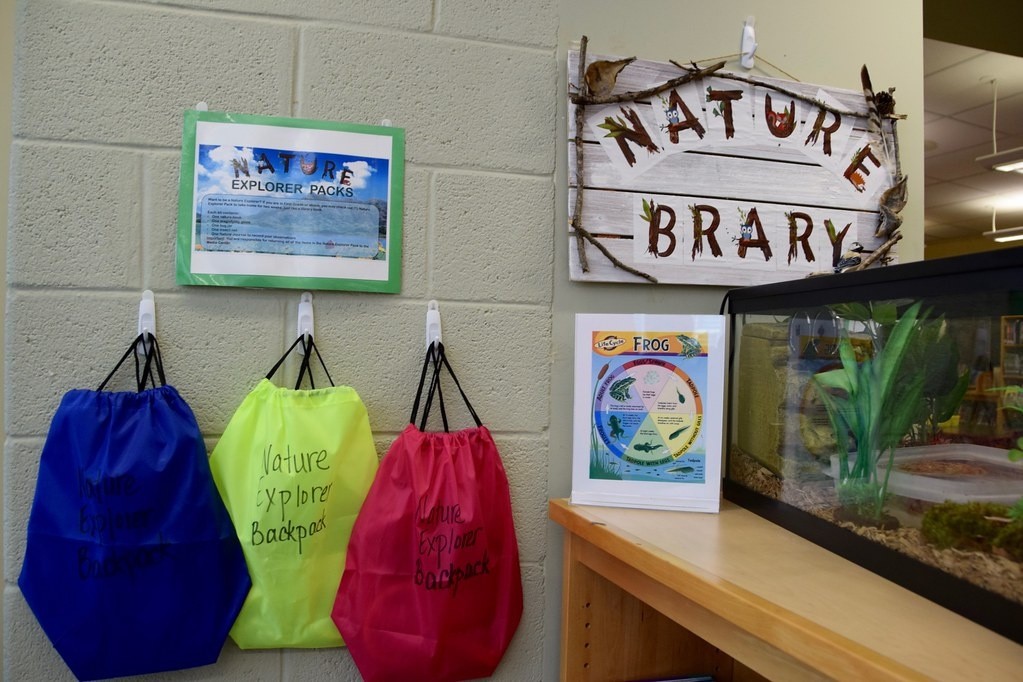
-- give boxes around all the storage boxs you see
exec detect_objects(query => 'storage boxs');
[721,243,1023,643]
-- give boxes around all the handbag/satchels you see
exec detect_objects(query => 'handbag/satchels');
[330,342,525,682]
[208,333,378,650]
[19,333,253,682]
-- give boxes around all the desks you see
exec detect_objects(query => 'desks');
[547,496,1023,681]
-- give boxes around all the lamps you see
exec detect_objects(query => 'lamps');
[974,76,1023,174]
[981,207,1023,243]
